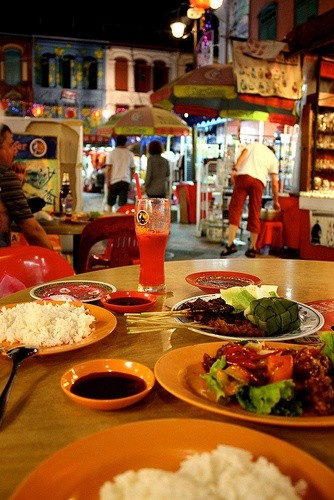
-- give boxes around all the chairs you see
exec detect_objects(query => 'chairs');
[0,205,151,298]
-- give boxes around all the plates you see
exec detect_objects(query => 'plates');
[60,359,156,411]
[154,341,334,428]
[8,418,334,500]
[29,279,116,303]
[102,291,157,314]
[171,294,325,342]
[0,300,117,359]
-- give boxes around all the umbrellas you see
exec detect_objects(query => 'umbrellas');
[99,105,190,178]
[150,63,299,187]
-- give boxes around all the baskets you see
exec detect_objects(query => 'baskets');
[261,207,282,221]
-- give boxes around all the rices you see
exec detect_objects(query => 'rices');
[99,443,307,500]
[0,299,95,348]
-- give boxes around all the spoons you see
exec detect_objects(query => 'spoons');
[0,345,38,427]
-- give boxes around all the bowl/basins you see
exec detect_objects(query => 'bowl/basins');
[185,271,262,295]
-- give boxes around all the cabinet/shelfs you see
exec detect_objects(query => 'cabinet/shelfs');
[301,93,334,192]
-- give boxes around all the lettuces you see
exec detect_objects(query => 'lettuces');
[199,331,334,417]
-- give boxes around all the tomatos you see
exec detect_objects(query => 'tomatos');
[265,355,294,382]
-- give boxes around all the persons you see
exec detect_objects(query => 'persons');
[0,124,172,253]
[219,143,281,256]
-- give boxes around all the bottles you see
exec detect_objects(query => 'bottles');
[65,190,74,221]
[59,172,70,218]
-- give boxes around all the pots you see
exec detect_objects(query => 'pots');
[259,208,281,221]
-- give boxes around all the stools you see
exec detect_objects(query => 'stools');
[260,220,284,246]
[171,204,180,224]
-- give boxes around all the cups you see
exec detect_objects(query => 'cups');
[134,198,171,293]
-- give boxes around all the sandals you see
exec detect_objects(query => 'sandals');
[220,243,237,256]
[245,248,256,258]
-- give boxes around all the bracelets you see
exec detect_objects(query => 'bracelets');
[232,167,238,172]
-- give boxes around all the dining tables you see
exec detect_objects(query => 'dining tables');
[0,259,334,500]
[44,224,84,273]
